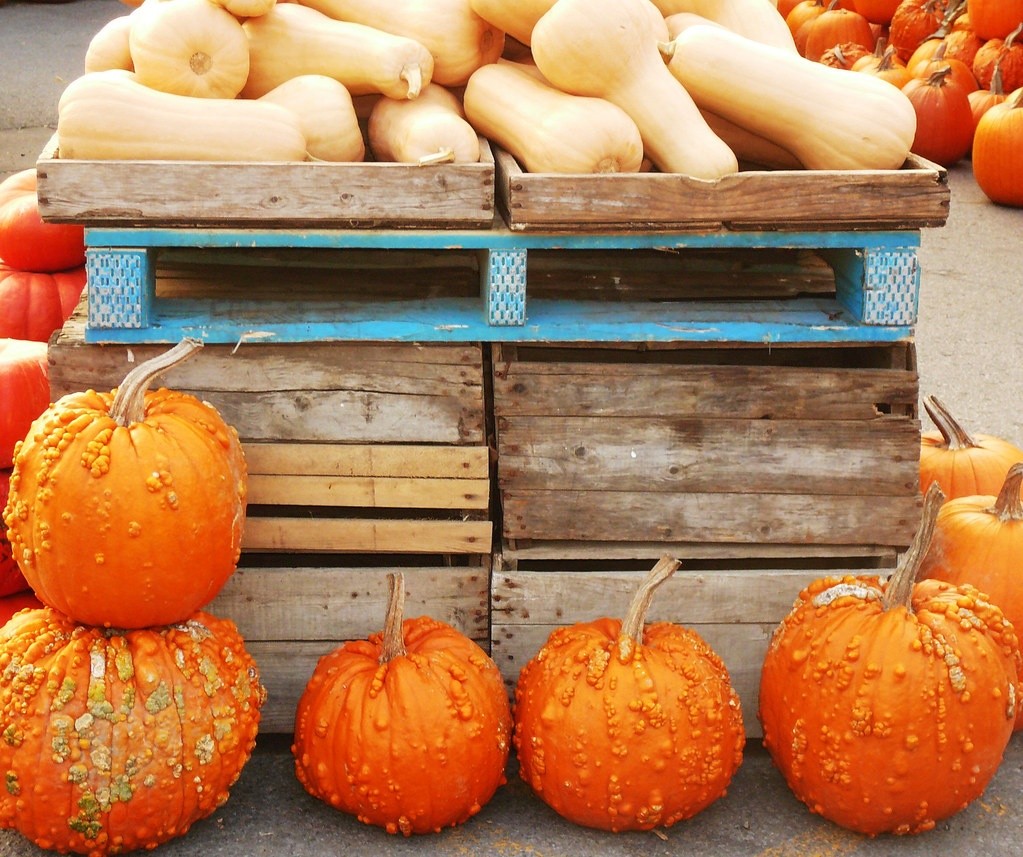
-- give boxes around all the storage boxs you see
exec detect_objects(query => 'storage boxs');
[493,341,924,549]
[491,533,904,742]
[492,143,954,233]
[35,125,499,232]
[197,554,491,736]
[47,279,497,561]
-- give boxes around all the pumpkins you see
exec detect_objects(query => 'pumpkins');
[0,1,1023,857]
